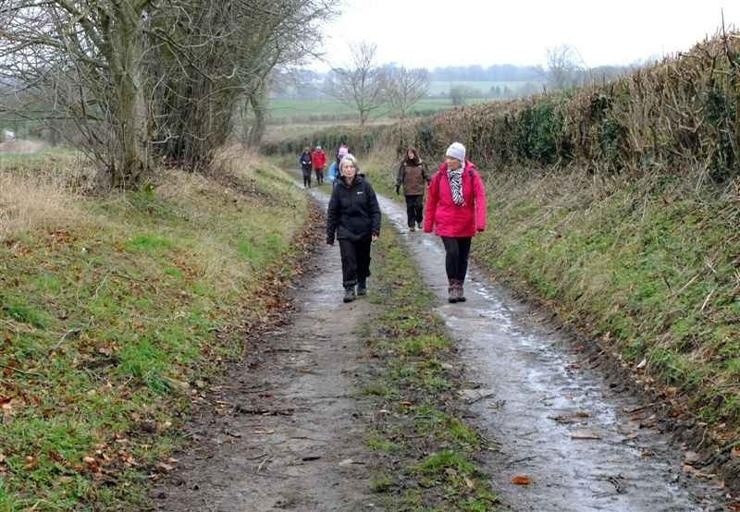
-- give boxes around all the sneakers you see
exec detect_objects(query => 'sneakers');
[344,286,356,301]
[418,223,422,228]
[356,279,367,296]
[410,225,415,231]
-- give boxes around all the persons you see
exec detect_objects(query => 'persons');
[326,153,382,303]
[328,155,343,192]
[424,142,488,303]
[335,140,351,160]
[299,146,313,189]
[396,146,432,232]
[311,145,328,185]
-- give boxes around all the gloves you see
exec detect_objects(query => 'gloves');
[396,186,400,195]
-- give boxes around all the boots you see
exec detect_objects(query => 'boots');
[448,279,465,303]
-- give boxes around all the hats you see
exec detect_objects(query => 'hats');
[445,141,466,161]
